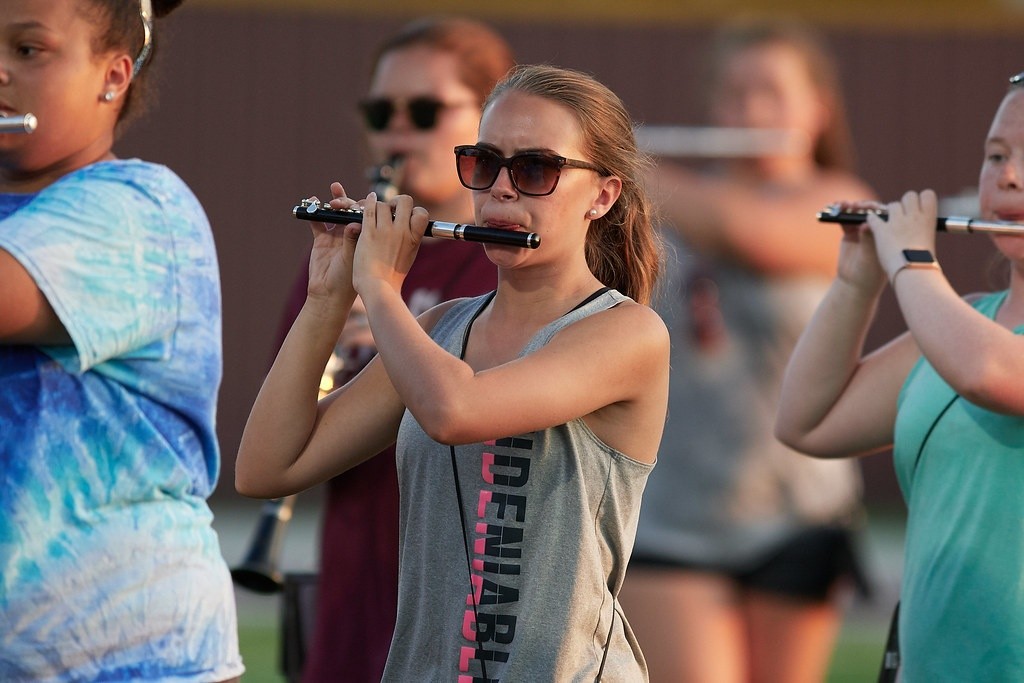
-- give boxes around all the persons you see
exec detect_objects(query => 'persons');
[235,65,670,683]
[0,0,246,683]
[271,19,514,683]
[774,73,1024,683]
[616,13,880,683]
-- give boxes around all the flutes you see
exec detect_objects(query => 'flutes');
[814,204,1024,239]
[290,197,543,250]
[0,110,40,136]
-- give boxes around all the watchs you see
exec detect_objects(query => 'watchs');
[888,249,939,286]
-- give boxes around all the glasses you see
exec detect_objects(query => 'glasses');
[454,145,609,196]
[356,98,480,131]
